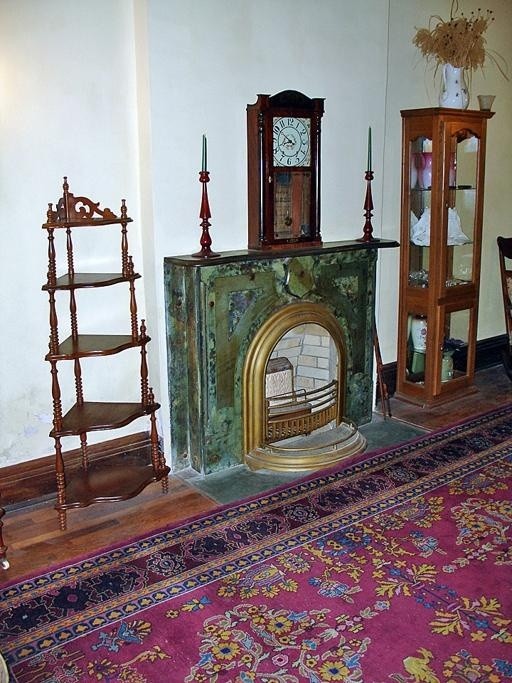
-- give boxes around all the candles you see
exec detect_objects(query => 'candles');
[202,132,209,173]
[367,124,374,173]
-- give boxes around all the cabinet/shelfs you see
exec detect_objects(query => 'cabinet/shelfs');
[395,104,496,410]
[40,175,173,530]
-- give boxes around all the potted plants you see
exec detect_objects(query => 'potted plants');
[411,0,511,109]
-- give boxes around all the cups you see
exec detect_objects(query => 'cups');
[408,268,458,291]
[476,94,497,112]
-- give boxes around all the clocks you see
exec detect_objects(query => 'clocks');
[244,89,326,253]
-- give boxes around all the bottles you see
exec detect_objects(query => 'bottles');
[410,151,456,187]
[410,313,427,351]
[442,351,456,383]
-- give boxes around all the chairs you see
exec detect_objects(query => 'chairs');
[494,234,512,354]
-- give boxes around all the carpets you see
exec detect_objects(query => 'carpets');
[0,397,512,681]
